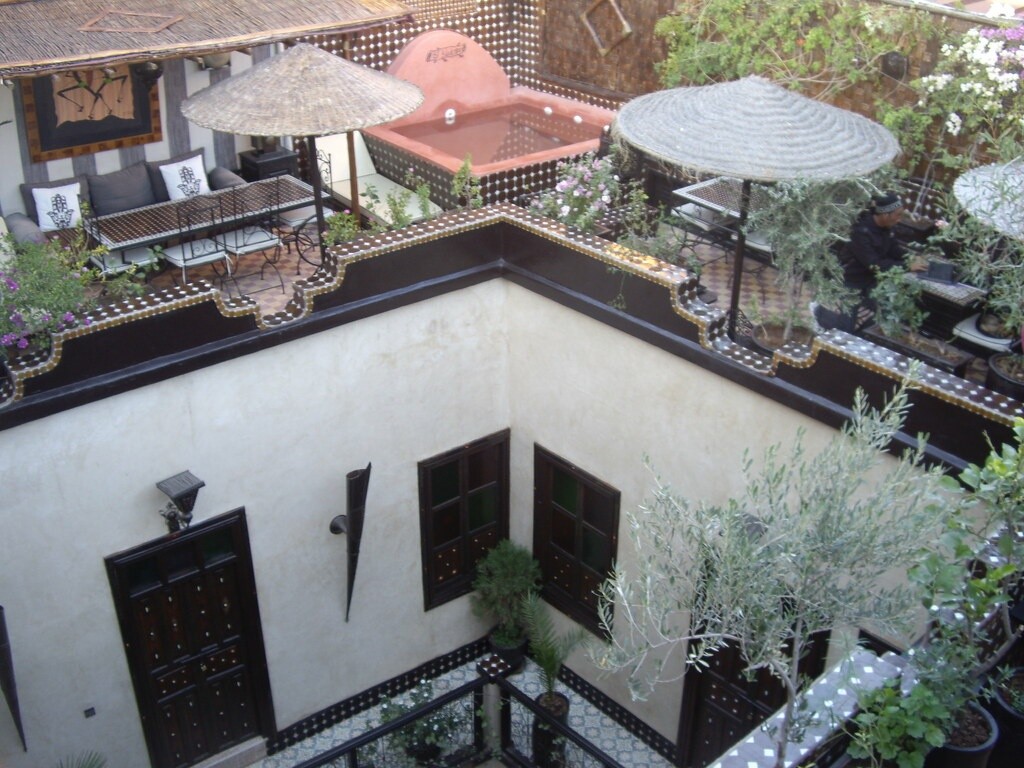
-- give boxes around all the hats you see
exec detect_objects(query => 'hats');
[871,189,903,213]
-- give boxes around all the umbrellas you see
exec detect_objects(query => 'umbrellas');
[179,41,423,265]
[616,74,902,341]
[951,159,1024,244]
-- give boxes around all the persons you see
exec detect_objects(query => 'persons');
[843,192,932,310]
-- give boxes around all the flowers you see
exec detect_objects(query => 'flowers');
[377,679,475,752]
[320,152,484,248]
[0,201,166,361]
[529,148,622,235]
[875,0,1024,223]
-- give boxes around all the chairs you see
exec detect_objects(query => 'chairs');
[670,201,736,266]
[953,313,1024,352]
[76,193,158,296]
[732,230,783,306]
[303,130,443,233]
[273,147,335,254]
[212,170,285,296]
[161,195,232,300]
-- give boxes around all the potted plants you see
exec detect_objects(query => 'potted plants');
[860,265,975,379]
[744,166,891,358]
[908,414,1024,768]
[517,589,594,768]
[468,539,543,675]
[985,296,1024,404]
[616,180,706,288]
[907,623,1024,768]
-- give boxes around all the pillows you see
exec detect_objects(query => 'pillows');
[32,181,83,233]
[20,174,95,227]
[86,161,157,216]
[158,154,211,201]
[144,146,206,202]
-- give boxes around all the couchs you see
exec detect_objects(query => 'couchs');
[4,166,248,270]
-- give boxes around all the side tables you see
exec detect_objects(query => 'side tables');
[238,146,300,229]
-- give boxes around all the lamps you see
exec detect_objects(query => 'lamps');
[156,469,206,533]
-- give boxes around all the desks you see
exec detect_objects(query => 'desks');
[903,270,987,348]
[673,176,795,222]
[83,173,332,291]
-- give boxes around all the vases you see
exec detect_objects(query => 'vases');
[406,742,442,762]
[591,221,614,242]
[892,220,937,246]
[5,327,49,360]
